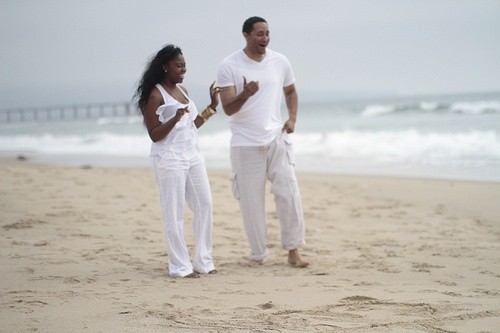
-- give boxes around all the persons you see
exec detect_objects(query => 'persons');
[139,45,222,278]
[217,16,309,267]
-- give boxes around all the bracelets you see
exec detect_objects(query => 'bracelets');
[200,106,215,120]
